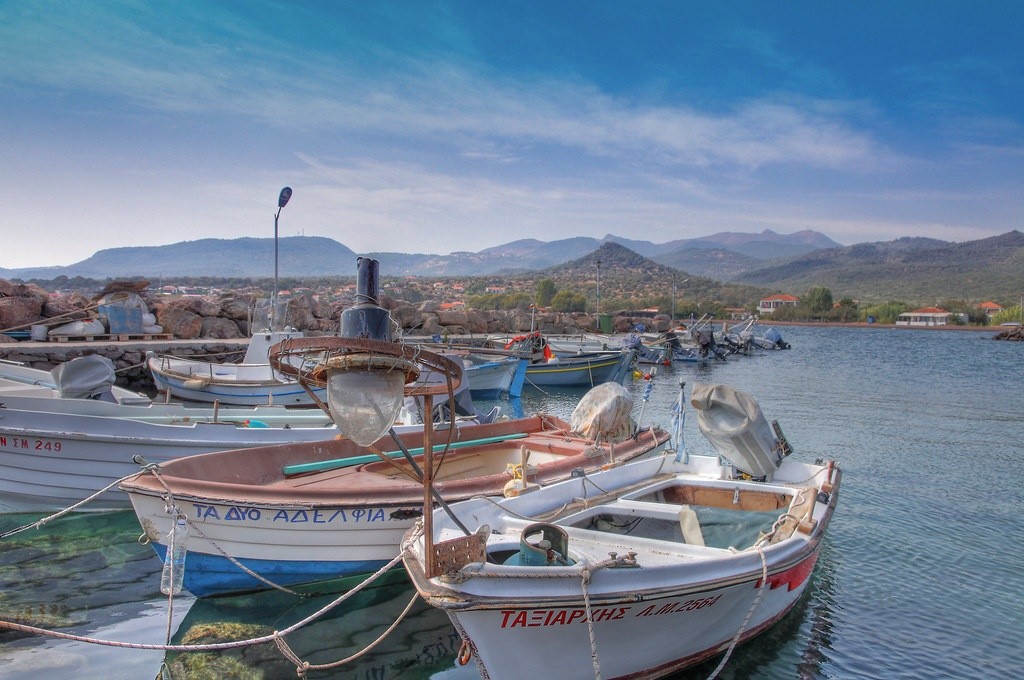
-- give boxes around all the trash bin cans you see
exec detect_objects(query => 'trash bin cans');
[599,315,614,334]
[868,316,874,324]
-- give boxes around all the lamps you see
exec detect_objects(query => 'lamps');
[267,256,486,580]
[671,377,688,465]
[634,366,657,438]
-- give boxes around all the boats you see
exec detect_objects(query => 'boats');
[484,312,791,386]
[0,356,512,514]
[147,298,469,416]
[401,380,844,680]
[118,379,674,601]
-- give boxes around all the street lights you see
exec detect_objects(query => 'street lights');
[597,260,601,329]
[275,186,292,307]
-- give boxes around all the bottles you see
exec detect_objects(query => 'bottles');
[159,519,188,595]
[244,419,271,428]
